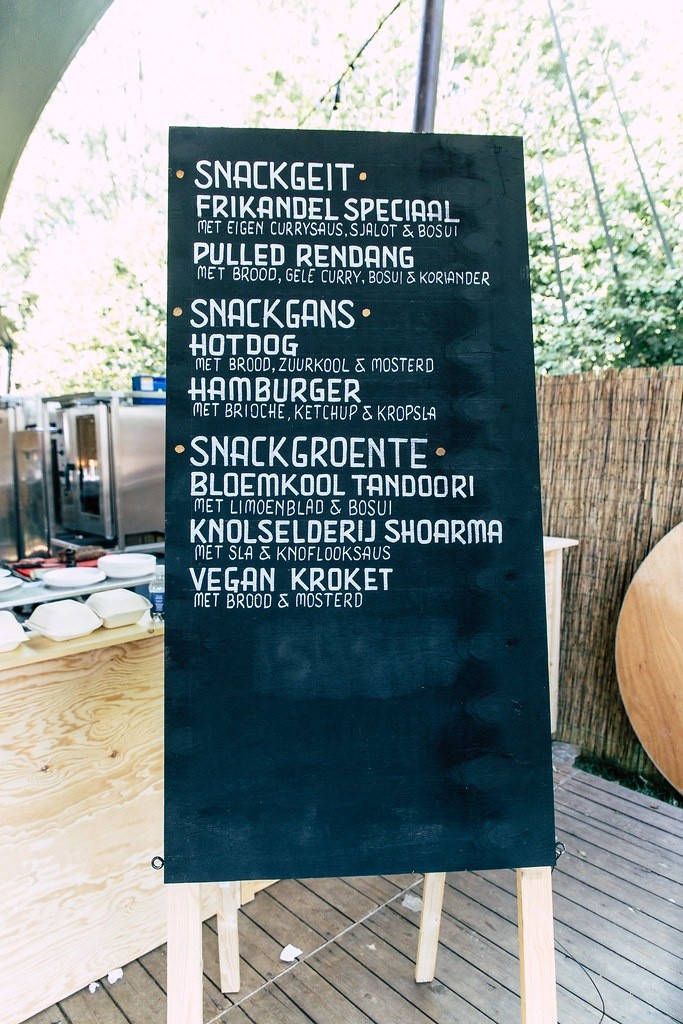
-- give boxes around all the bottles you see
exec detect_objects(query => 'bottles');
[148,564,164,624]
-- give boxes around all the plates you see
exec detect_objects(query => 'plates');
[42,566,105,588]
[0,568,11,577]
[98,552,156,578]
[0,577,23,592]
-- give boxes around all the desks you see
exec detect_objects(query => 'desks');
[0,563,165,609]
